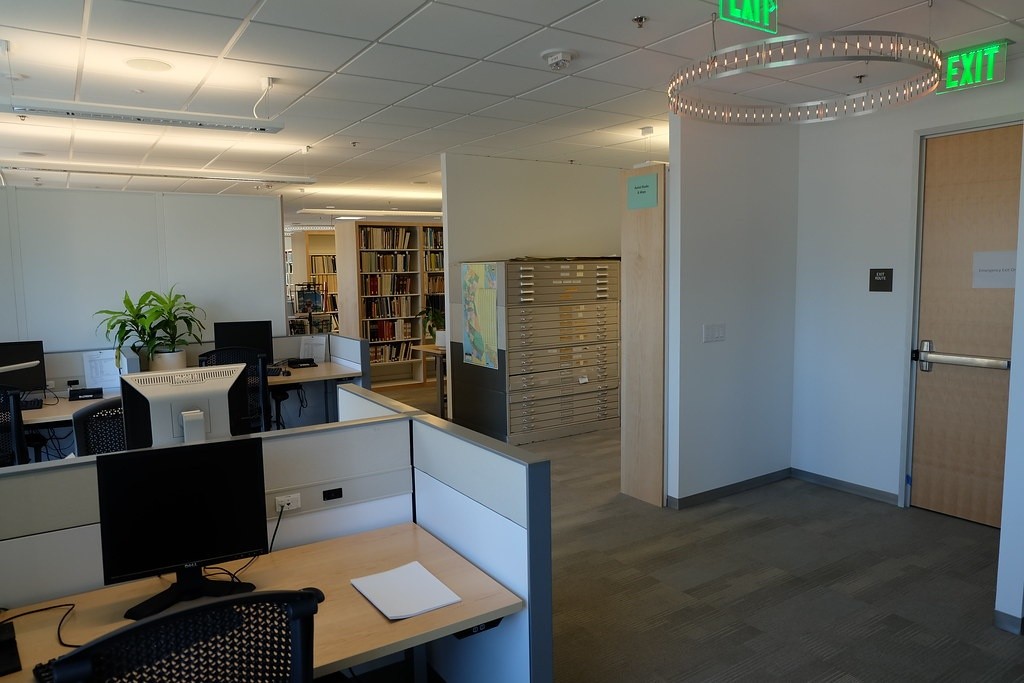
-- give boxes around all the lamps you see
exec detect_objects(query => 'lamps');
[1,39,284,134]
[665,0,941,128]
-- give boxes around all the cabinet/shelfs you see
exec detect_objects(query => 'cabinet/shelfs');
[303,229,337,333]
[334,218,445,389]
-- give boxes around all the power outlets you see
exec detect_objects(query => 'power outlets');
[275,492,302,513]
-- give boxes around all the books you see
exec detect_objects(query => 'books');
[363,319,411,342]
[310,275,339,331]
[363,296,410,318]
[423,251,444,271]
[427,275,445,314]
[361,251,410,272]
[310,255,336,273]
[359,226,410,249]
[369,342,413,362]
[362,274,410,296]
[424,227,444,249]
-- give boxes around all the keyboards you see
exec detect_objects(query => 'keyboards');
[19,399,43,410]
[33,655,61,683]
[267,367,282,376]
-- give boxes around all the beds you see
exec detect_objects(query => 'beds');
[0,382,42,469]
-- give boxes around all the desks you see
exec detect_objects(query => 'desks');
[0,335,555,683]
[410,344,446,420]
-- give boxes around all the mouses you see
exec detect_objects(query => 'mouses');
[283,370,291,377]
[297,587,325,603]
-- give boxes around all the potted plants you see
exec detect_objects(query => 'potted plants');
[90,282,206,372]
[413,306,446,347]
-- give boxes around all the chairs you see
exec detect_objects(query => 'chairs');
[33,587,325,683]
[71,395,124,457]
[197,345,269,437]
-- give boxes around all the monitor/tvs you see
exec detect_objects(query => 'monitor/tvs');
[94,319,274,621]
[0,341,46,392]
